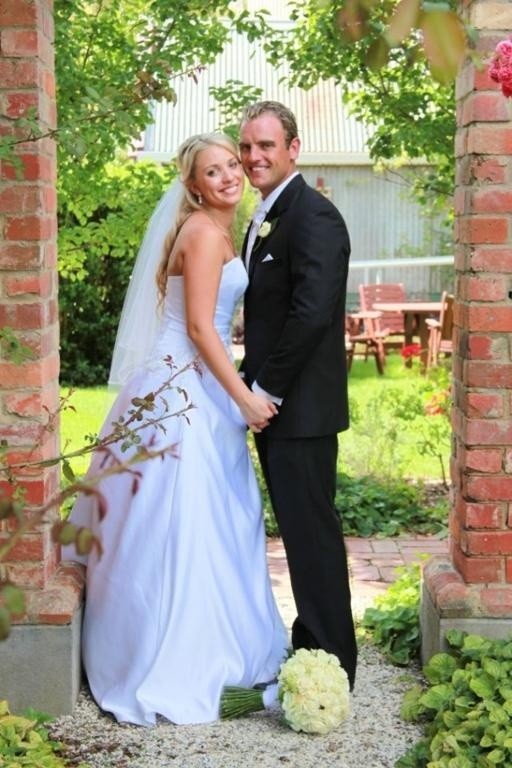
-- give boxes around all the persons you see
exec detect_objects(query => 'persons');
[61,135,289,730]
[231,99,361,702]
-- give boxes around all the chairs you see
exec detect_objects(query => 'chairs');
[343,284,456,375]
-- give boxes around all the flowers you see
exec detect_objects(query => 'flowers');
[488,37,512,94]
[257,221,271,237]
[219,658,352,739]
